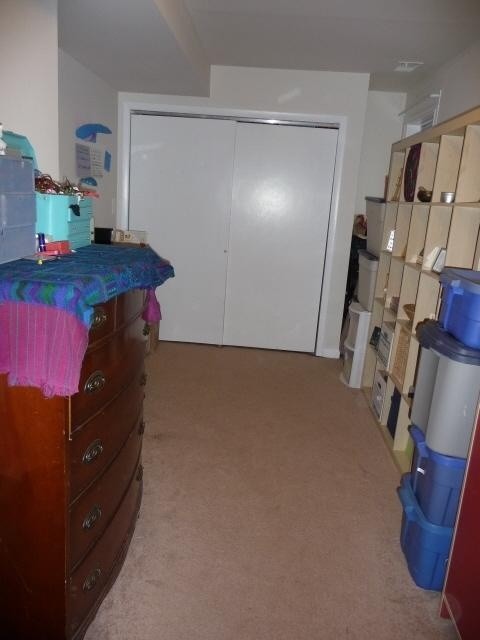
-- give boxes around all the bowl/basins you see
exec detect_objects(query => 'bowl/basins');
[417,184,432,202]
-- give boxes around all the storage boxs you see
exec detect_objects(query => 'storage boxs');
[339,340,366,392]
[392,470,456,593]
[356,249,376,312]
[403,423,468,527]
[364,195,387,260]
[409,315,480,463]
[436,263,480,346]
[343,299,372,351]
[0,145,94,264]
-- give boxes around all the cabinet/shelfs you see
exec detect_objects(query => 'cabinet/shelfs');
[0,243,154,639]
[360,100,479,476]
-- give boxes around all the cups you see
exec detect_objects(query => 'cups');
[439,192,454,204]
